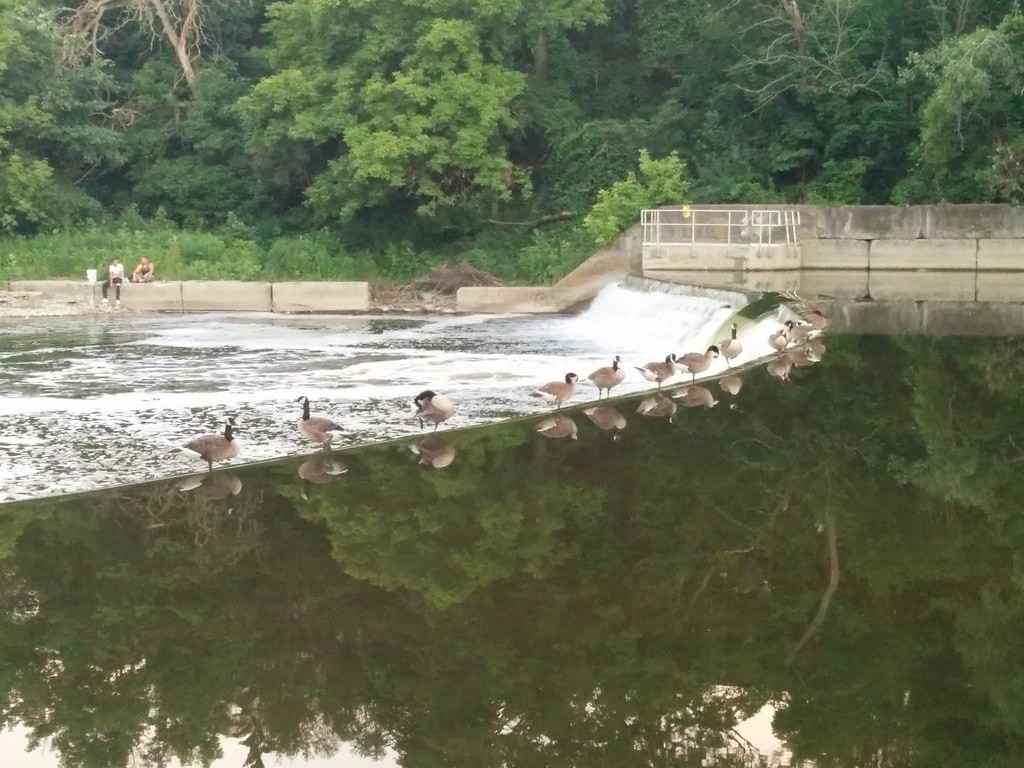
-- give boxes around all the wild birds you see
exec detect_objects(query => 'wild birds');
[415,391,456,434]
[529,373,578,409]
[582,405,628,441]
[293,397,344,453]
[674,345,719,383]
[407,437,456,471]
[634,353,678,388]
[717,375,743,411]
[788,351,814,367]
[635,392,677,424]
[765,357,793,382]
[534,413,578,442]
[181,418,241,470]
[587,356,626,399]
[718,323,743,370]
[767,320,817,351]
[670,385,719,410]
[296,454,351,501]
[806,341,826,362]
[175,472,242,520]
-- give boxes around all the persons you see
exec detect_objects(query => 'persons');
[131,257,154,283]
[100,259,123,305]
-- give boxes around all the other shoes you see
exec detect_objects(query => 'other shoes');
[116,300,121,304]
[102,298,109,302]
